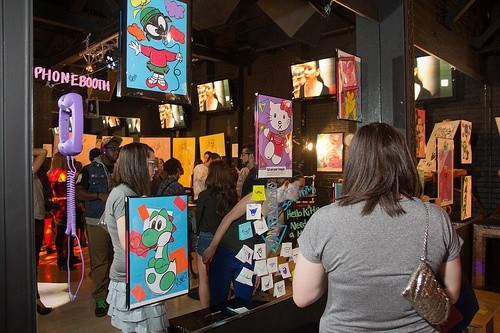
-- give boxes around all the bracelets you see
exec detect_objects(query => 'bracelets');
[97,192,101,200]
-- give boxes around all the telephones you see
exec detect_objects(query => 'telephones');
[58,95,69,156]
[64,93,83,155]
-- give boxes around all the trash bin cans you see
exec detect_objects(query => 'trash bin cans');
[472,216,500,293]
[449,212,471,229]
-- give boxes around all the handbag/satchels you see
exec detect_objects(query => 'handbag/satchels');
[401,200,454,328]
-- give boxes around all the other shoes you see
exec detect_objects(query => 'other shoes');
[36,303,53,315]
[46,247,55,254]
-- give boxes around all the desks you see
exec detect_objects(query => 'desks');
[467,289,500,333]
[471,212,500,289]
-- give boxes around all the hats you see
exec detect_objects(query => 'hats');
[101,137,122,149]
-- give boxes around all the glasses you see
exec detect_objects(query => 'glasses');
[146,158,157,167]
[104,145,119,151]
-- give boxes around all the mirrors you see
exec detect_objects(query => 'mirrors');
[31,0,382,333]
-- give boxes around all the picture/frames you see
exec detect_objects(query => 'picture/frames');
[116,0,194,106]
[434,136,455,208]
[334,48,362,123]
[315,132,344,174]
[254,93,295,180]
[123,195,191,309]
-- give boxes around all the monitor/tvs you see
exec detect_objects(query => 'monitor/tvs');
[195,76,235,113]
[414,53,456,103]
[156,103,188,130]
[289,56,338,100]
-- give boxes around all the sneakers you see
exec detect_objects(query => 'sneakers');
[95,298,109,318]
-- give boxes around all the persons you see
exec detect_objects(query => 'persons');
[45,148,249,271]
[241,144,267,199]
[106,142,169,333]
[201,82,223,110]
[195,158,237,308]
[202,170,305,305]
[298,60,329,97]
[158,157,185,196]
[134,118,140,132]
[161,104,178,129]
[108,117,118,127]
[74,136,123,317]
[413,57,431,100]
[192,153,221,202]
[292,122,464,333]
[32,149,54,315]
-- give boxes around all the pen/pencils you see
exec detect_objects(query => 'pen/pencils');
[205,311,221,318]
[227,307,233,311]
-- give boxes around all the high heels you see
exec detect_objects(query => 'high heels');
[56,256,76,272]
[69,256,82,263]
